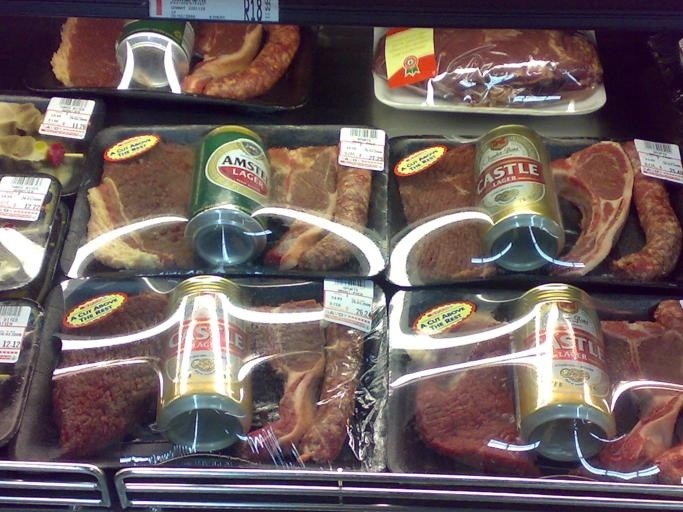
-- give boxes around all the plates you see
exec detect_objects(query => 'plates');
[371,27,608,115]
[1,169,63,300]
[385,133,683,292]
[1,296,45,447]
[14,277,387,472]
[386,288,683,484]
[20,15,335,112]
[59,124,392,278]
[1,139,84,199]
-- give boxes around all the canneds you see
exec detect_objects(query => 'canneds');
[115,18,195,87]
[158,275,252,452]
[511,282,616,462]
[185,125,271,267]
[474,124,565,271]
